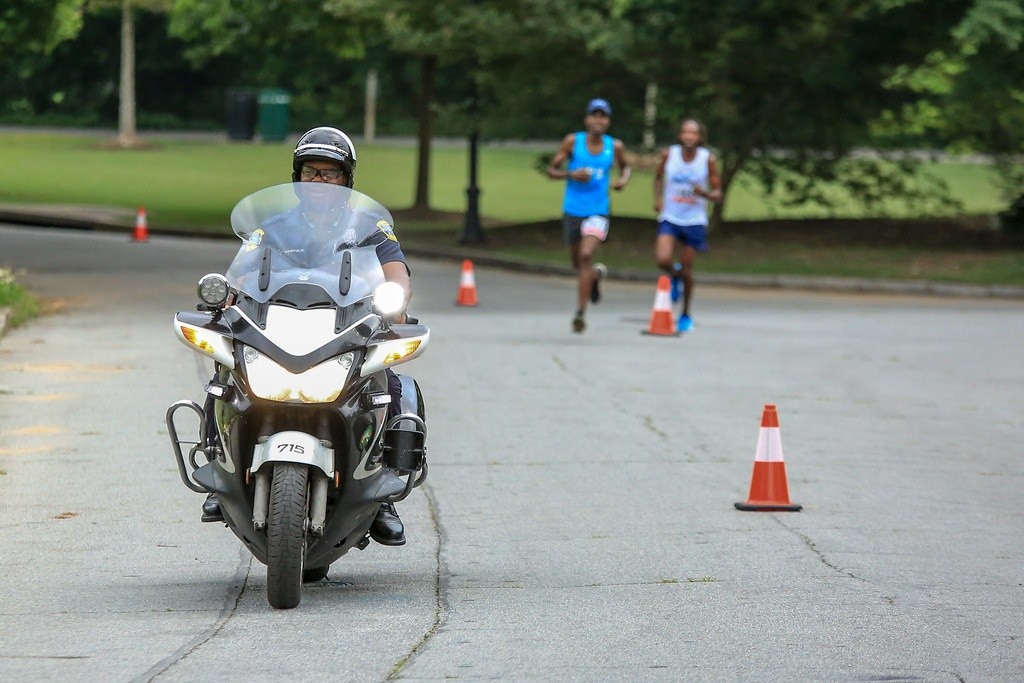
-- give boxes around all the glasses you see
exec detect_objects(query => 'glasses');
[302,163,342,181]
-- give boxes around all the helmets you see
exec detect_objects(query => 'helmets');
[291,126,356,201]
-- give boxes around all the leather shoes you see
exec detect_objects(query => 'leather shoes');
[201,491,224,522]
[370,502,406,546]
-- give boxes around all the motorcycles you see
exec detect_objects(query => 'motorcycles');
[161,179,432,612]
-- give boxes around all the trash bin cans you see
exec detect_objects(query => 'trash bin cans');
[257,79,292,143]
[223,89,257,141]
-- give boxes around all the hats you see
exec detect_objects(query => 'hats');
[589,99,612,116]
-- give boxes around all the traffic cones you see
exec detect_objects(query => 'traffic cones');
[127,206,152,245]
[733,403,802,512]
[640,275,682,336]
[453,259,481,307]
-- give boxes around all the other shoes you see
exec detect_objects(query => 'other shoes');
[574,317,585,332]
[590,263,606,303]
[678,314,692,332]
[672,263,683,303]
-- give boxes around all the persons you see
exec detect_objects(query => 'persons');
[199,126,413,547]
[652,118,722,334]
[546,99,632,333]
